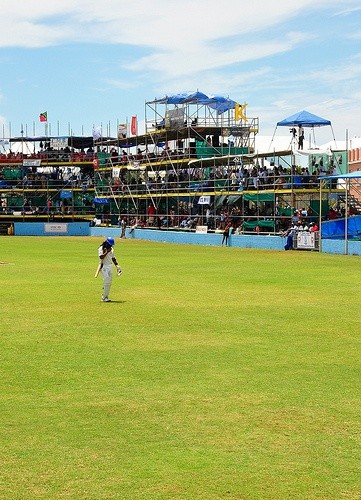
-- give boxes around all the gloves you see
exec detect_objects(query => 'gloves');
[116,265,122,276]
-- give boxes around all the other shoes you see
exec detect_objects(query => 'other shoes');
[101,294,103,298]
[101,298,111,302]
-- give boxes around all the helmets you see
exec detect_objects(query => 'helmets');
[107,237,114,245]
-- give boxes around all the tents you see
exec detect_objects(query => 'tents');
[160,92,242,125]
[267,110,337,154]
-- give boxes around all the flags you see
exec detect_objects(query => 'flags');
[40,111,47,121]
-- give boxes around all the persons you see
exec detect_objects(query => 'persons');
[97,236,120,303]
[0,118,361,251]
